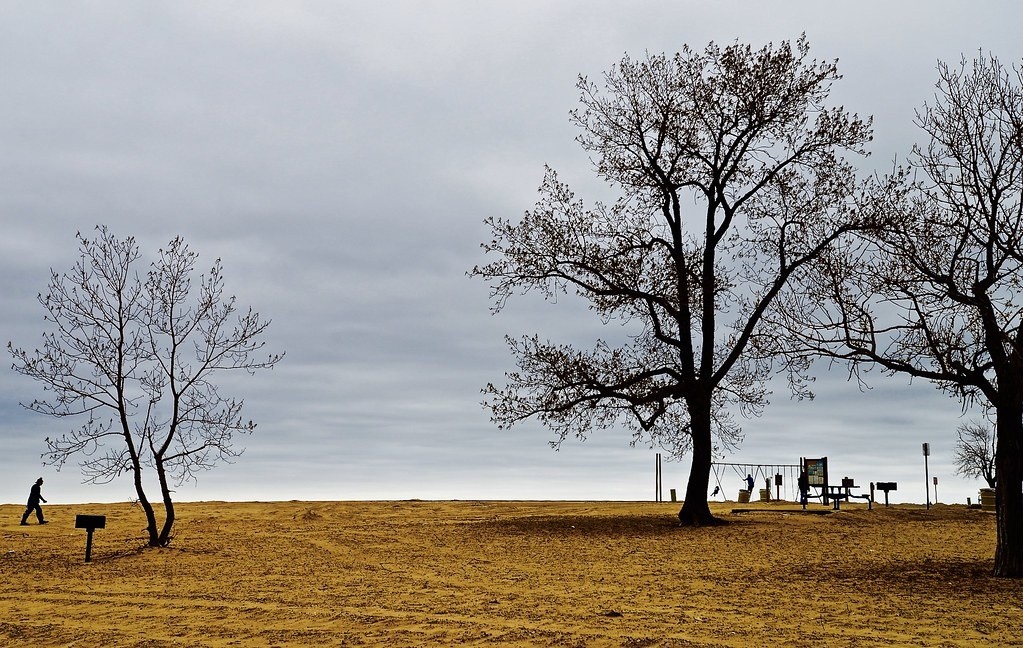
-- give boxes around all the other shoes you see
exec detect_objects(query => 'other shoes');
[40,520,49,524]
[21,521,30,525]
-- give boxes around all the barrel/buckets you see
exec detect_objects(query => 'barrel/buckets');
[738,488,750,503]
[759,489,770,501]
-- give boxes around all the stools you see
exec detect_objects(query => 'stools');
[826,493,873,511]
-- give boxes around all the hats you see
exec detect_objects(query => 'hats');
[35,478,43,483]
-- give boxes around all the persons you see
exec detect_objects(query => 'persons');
[19,477,49,526]
[710,486,719,496]
[742,474,755,496]
[798,472,810,505]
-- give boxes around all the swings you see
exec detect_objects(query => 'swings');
[732,465,754,487]
[714,464,725,494]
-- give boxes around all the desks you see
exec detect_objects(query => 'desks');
[829,486,860,493]
